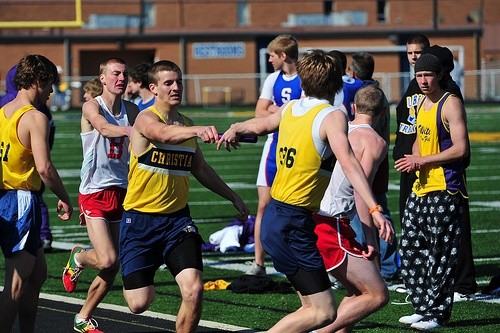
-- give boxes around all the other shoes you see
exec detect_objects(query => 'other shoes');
[453,291,476,302]
[398,313,441,331]
[231,260,267,292]
[43,239,52,251]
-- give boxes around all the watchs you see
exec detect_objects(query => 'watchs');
[368,204,383,214]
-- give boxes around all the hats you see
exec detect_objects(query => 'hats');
[415,54,442,74]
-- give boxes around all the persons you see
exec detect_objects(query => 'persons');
[313,86,388,333]
[476,266,500,303]
[324,50,379,122]
[452,48,463,87]
[60,58,141,333]
[119,60,250,333]
[392,32,475,302]
[246,33,306,277]
[394,54,466,330]
[217,49,395,333]
[81,62,169,269]
[0,54,73,333]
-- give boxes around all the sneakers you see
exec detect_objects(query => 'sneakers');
[62,246,86,293]
[73,314,104,333]
